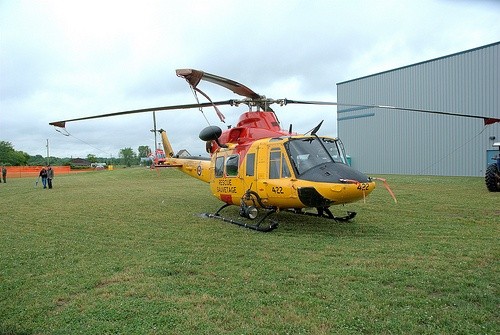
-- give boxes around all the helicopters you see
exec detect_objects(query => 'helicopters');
[47,67,500,231]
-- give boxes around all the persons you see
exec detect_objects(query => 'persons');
[38,167,54,189]
[0,165,7,183]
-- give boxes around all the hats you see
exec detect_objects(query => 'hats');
[46,164,50,167]
